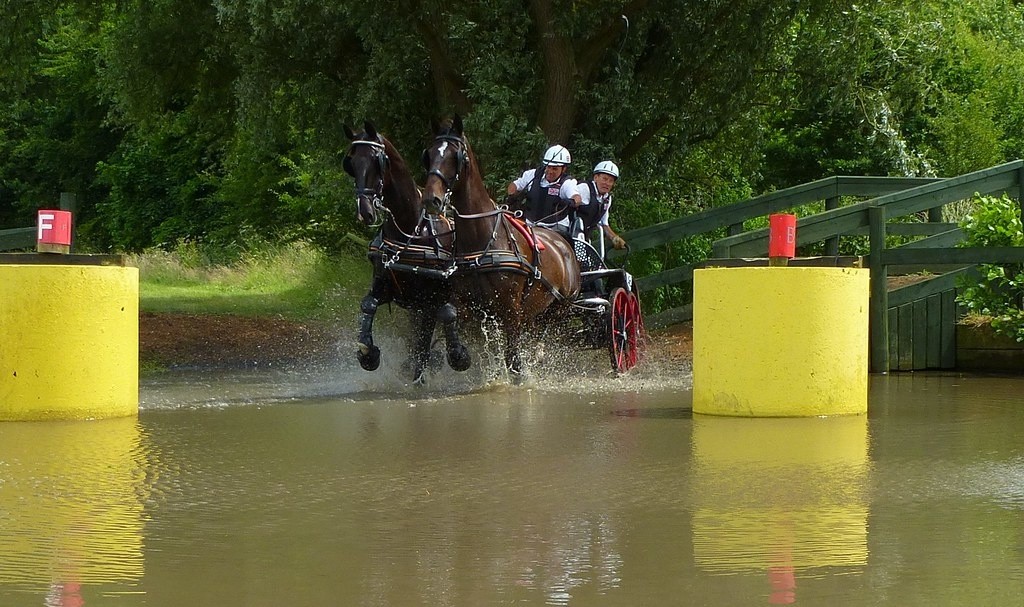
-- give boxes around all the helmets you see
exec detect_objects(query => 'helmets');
[543,144,572,166]
[593,161,619,181]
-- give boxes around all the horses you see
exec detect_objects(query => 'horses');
[339,113,582,390]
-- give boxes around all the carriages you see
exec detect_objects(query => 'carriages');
[341,113,646,389]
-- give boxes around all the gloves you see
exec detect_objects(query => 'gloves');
[613,235,627,249]
[507,194,520,205]
[562,198,575,211]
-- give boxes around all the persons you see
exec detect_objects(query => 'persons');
[576,161,626,296]
[508,145,581,251]
